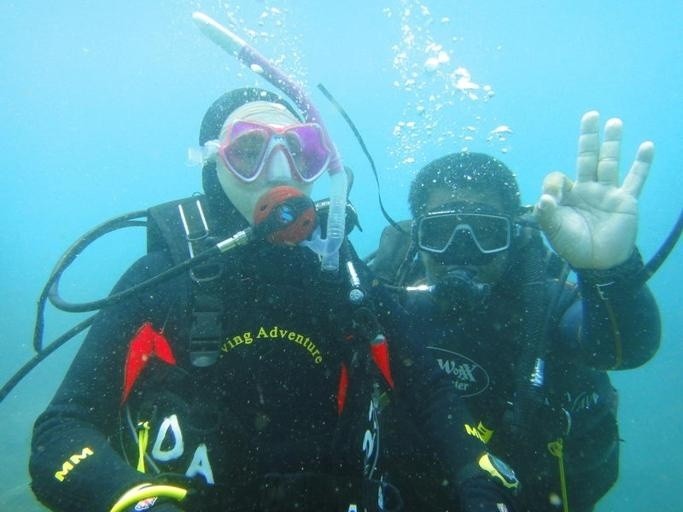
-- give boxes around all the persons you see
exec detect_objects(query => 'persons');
[366,109,662,512]
[28,86,525,512]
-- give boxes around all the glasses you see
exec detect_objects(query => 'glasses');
[414,209,514,255]
[217,117,333,183]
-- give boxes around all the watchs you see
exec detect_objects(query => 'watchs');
[448,448,523,496]
